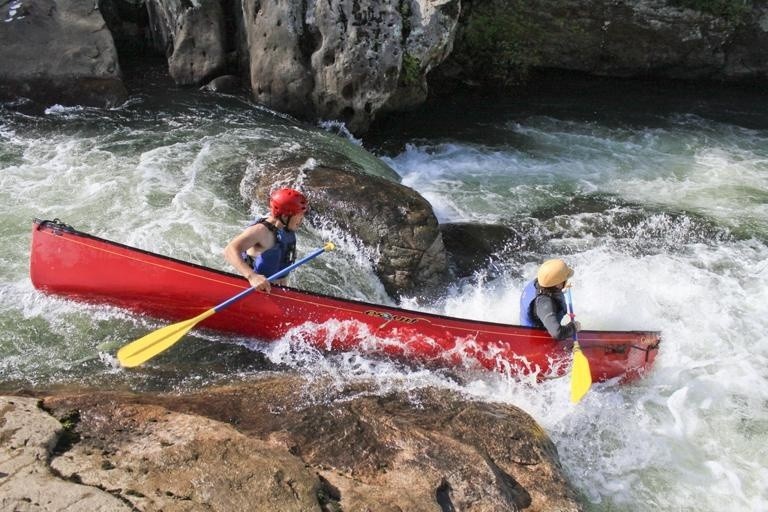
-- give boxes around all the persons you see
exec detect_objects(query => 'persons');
[519,259,582,342]
[224,187,308,295]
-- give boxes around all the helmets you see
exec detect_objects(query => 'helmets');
[538,259,574,288]
[270,188,308,218]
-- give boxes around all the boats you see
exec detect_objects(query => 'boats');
[24,216,662,398]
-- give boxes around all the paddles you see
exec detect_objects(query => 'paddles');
[117,241,334,367]
[566,286,592,401]
